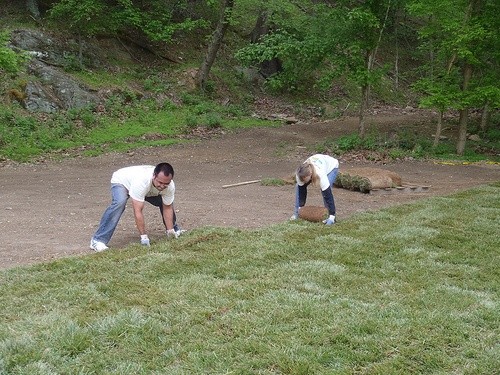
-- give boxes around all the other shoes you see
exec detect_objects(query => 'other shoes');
[290,215,296,221]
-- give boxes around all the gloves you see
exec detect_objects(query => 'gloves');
[167,228,179,239]
[140,234,151,247]
[323,215,336,225]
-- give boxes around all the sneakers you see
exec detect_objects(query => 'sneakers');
[90,238,109,253]
[176,229,187,236]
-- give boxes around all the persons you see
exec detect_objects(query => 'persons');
[89,162,187,254]
[288,154,340,225]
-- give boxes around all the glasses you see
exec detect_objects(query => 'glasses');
[154,178,170,186]
